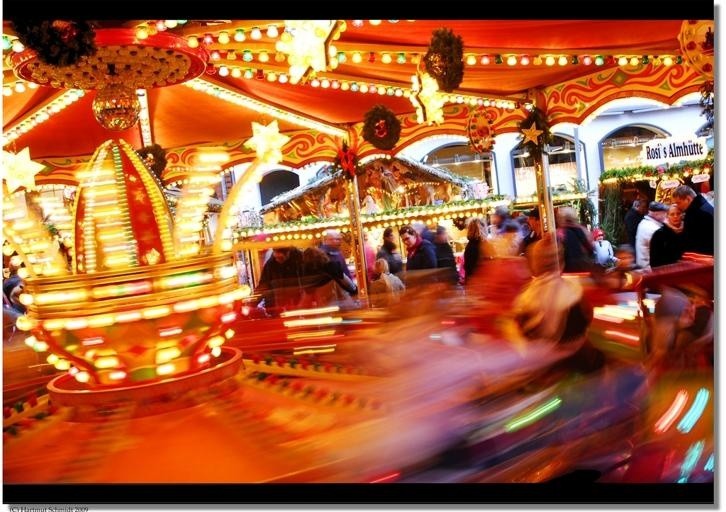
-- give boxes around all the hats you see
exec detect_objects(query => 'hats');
[523,205,539,217]
[593,229,604,240]
[373,259,388,273]
[649,201,669,212]
[487,205,510,216]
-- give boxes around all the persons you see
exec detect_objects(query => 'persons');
[256,223,461,307]
[468,204,619,385]
[631,185,716,267]
